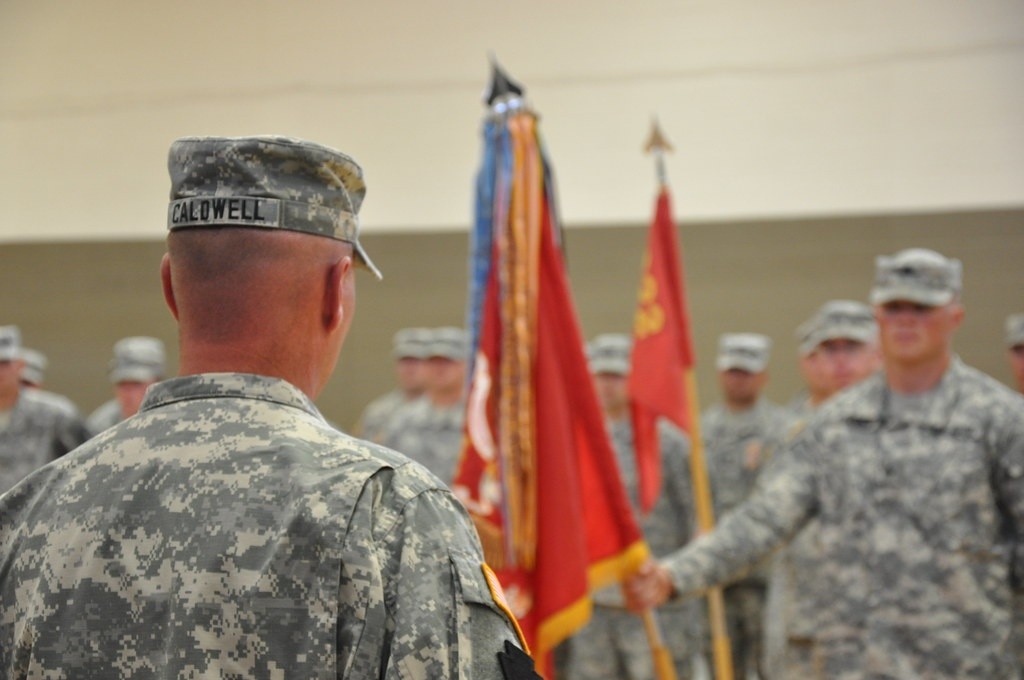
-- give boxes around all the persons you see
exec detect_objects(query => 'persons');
[1003,312,1024,396]
[347,298,879,680]
[0,320,170,494]
[621,248,1024,680]
[0,138,545,680]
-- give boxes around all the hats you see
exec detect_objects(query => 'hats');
[869,247,963,308]
[714,333,772,374]
[18,349,48,383]
[166,136,384,280]
[1007,313,1024,348]
[796,298,877,357]
[0,326,18,361]
[587,333,630,374]
[110,334,163,383]
[394,326,465,363]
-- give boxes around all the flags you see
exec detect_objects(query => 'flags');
[632,184,692,515]
[451,108,650,680]
